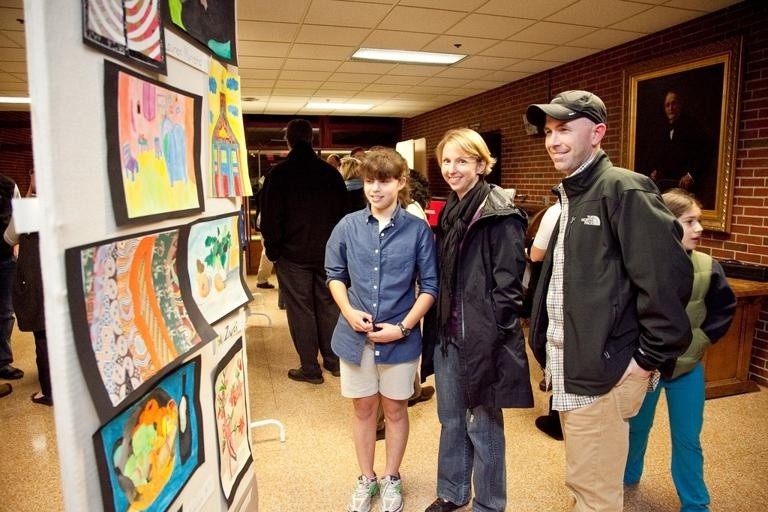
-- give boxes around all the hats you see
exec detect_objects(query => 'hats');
[526,89,607,129]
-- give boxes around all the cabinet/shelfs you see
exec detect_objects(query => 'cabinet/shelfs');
[691,272,768,401]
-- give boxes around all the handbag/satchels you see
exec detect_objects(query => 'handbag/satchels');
[12,274,39,332]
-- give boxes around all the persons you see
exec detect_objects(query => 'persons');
[252,120,368,385]
[522,88,694,512]
[421,128,529,512]
[624,186,738,511]
[0,173,24,401]
[325,146,439,512]
[648,86,713,206]
[3,170,53,408]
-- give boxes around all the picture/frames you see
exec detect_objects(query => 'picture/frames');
[611,29,749,239]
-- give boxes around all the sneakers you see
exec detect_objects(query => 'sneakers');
[346,471,379,512]
[256,281,275,289]
[378,474,405,512]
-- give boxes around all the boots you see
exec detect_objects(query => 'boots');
[535,395,564,440]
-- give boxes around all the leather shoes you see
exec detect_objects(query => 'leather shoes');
[323,362,340,377]
[288,367,323,384]
[375,423,385,440]
[425,496,470,511]
[408,385,435,406]
[539,378,552,392]
[0,364,24,398]
[31,391,53,407]
